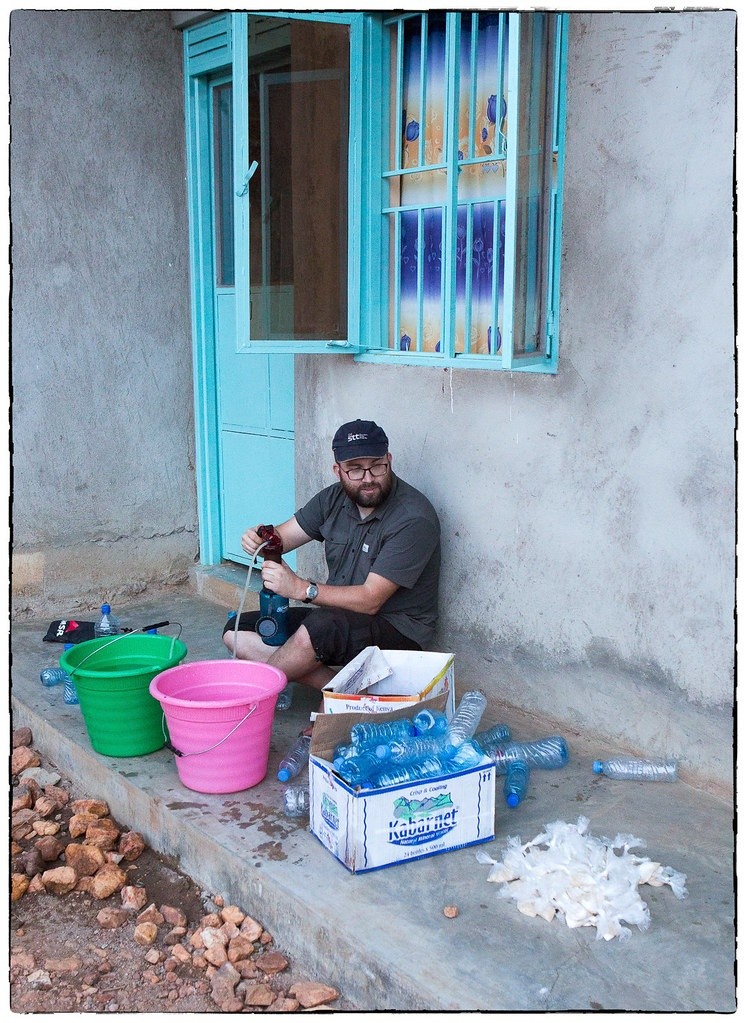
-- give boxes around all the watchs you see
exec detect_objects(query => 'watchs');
[302,582,319,604]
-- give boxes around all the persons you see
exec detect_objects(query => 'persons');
[222,419,442,737]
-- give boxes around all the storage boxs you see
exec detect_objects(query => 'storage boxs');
[307,692,496,877]
[309,646,456,726]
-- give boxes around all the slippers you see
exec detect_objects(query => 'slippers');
[298,721,314,744]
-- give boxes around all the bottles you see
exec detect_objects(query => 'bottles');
[147,628,158,635]
[281,784,310,817]
[593,757,679,784]
[62,643,80,704]
[94,604,121,638]
[40,668,67,687]
[255,580,288,646]
[277,735,311,782]
[276,681,294,712]
[332,688,567,808]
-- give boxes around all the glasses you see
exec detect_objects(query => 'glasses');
[336,460,389,481]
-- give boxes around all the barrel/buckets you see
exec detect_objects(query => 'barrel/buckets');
[60,619,187,758]
[148,658,287,795]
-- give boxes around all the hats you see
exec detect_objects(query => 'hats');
[332,419,390,463]
